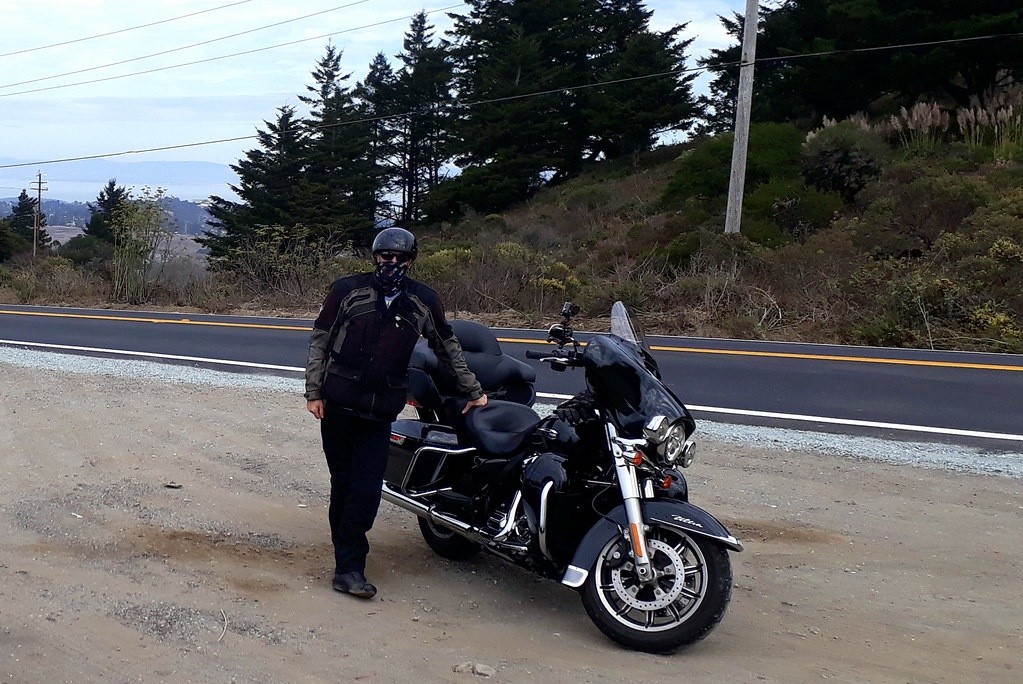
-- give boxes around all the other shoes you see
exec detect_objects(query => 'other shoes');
[333,571,377,598]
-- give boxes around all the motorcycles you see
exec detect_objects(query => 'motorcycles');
[380,296,744,658]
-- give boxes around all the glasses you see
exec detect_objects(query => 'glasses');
[378,250,411,262]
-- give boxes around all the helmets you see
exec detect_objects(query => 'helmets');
[372,227,418,260]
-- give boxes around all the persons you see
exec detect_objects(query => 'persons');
[305,228,487,599]
